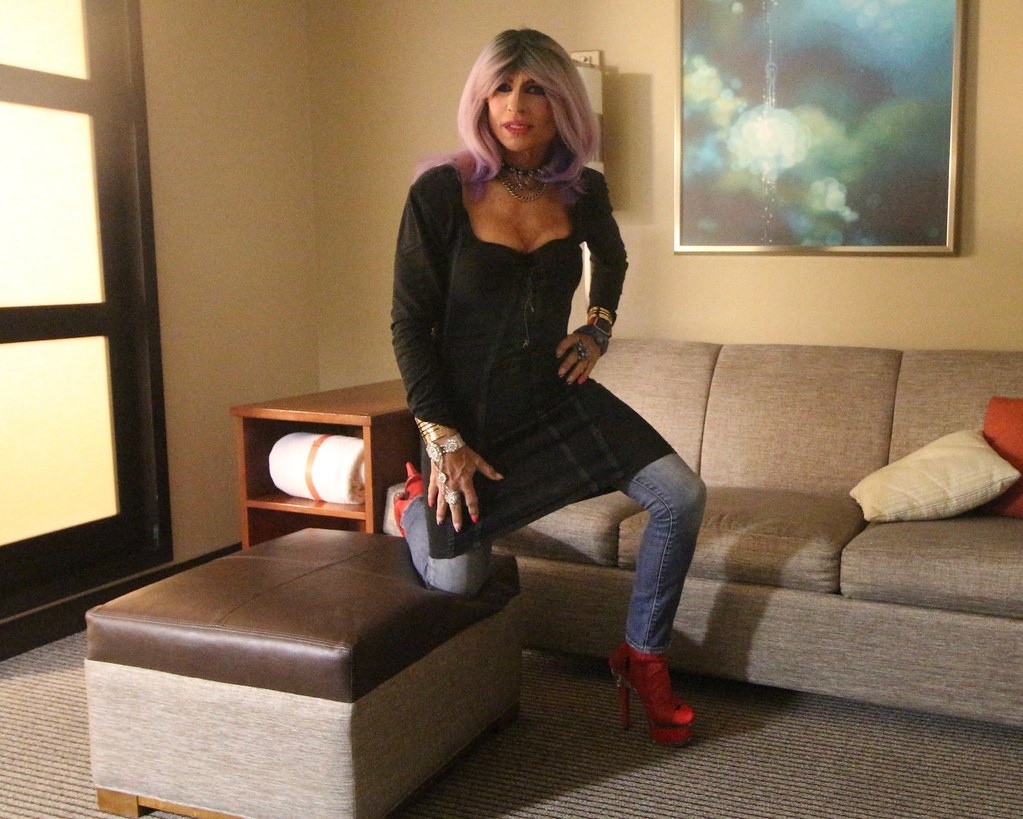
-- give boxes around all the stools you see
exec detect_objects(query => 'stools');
[82,528,525,819]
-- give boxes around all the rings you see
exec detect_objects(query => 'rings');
[573,339,589,361]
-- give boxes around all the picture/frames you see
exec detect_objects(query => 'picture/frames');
[674,0,964,256]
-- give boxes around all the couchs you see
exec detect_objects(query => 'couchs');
[383,338,1022,731]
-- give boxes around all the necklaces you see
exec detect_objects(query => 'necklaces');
[496,160,547,202]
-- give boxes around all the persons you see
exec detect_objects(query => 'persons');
[391,28,706,746]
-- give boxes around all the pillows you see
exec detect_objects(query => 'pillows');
[848,429,1021,523]
[978,397,1023,521]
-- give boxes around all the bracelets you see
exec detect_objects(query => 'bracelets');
[426,433,467,504]
[587,306,613,324]
[414,417,458,445]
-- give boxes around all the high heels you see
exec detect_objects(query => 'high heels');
[394,462,426,537]
[606,641,698,747]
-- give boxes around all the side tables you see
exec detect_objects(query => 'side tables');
[230,379,426,552]
